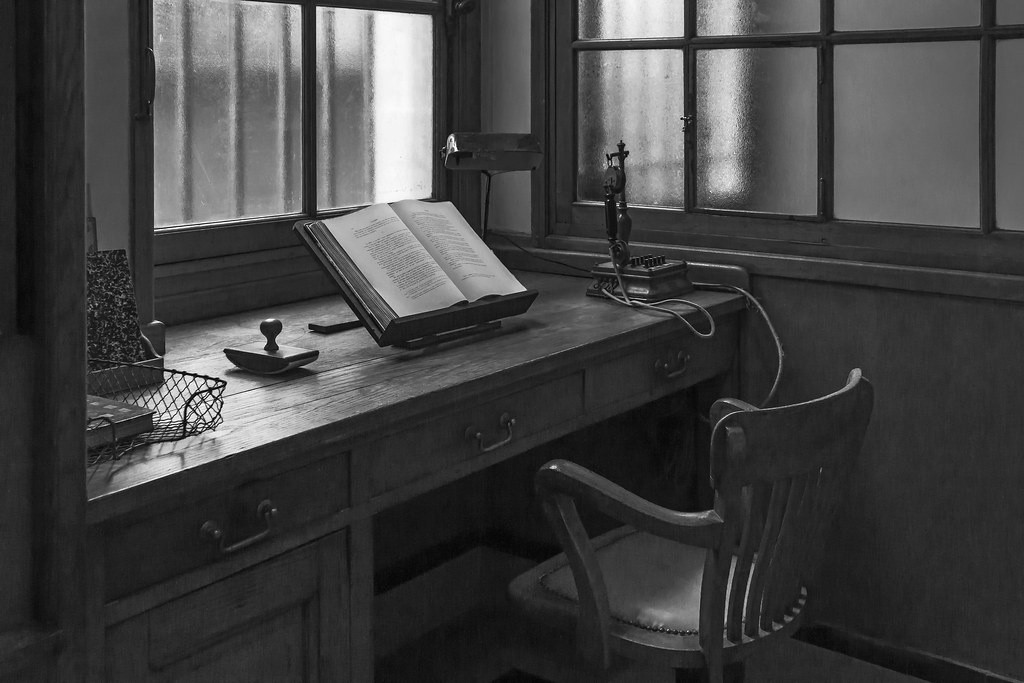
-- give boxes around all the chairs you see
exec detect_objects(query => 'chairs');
[508,368,871,683]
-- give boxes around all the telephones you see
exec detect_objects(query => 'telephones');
[586,139,695,303]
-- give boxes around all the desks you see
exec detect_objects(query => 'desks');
[86,246,750,683]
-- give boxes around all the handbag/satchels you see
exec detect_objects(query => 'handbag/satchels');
[87,219,149,367]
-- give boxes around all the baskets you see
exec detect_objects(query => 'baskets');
[85,355,227,467]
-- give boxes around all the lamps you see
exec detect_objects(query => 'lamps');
[439,133,545,241]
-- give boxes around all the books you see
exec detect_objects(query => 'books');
[305,200,527,332]
